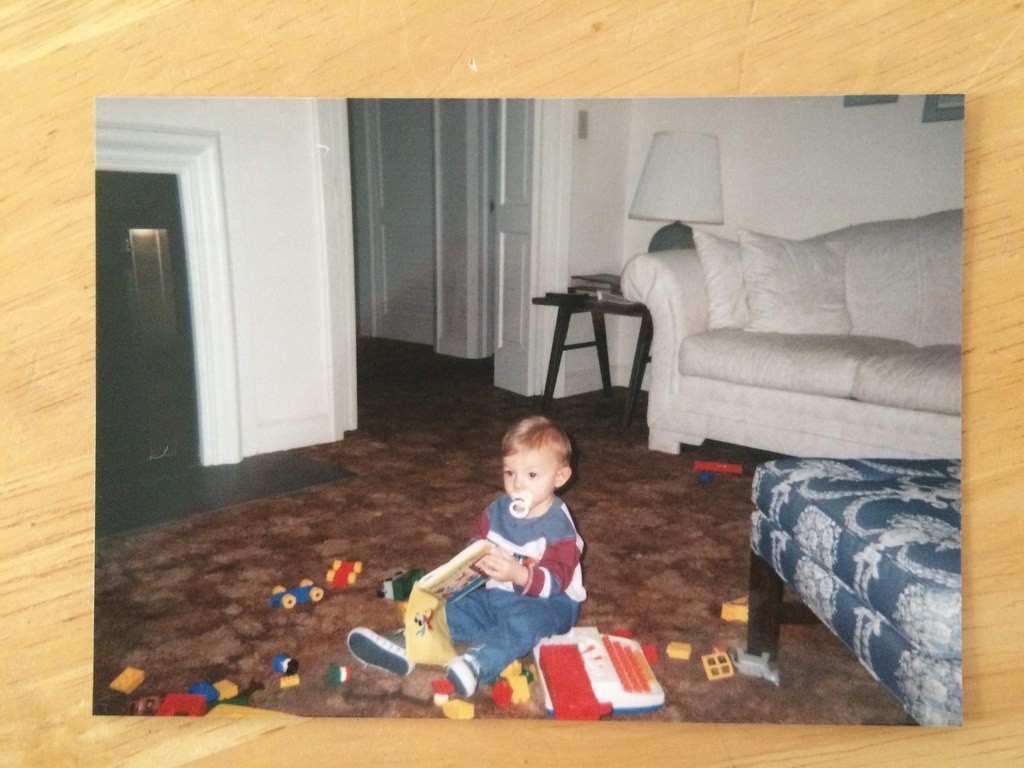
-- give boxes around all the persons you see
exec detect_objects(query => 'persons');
[343,414,588,700]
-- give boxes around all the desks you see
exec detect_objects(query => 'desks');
[531,292,654,428]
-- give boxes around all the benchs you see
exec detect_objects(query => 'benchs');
[747,458,963,727]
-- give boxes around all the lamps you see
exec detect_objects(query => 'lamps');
[628,131,725,253]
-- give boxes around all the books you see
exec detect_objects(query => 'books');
[571,273,634,305]
[417,540,492,603]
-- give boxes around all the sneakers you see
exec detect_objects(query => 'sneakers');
[346,626,418,677]
[444,652,480,699]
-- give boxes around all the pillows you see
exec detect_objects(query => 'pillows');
[692,228,749,331]
[737,227,852,336]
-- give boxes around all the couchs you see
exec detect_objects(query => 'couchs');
[618,206,962,462]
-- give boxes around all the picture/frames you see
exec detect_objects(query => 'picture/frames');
[922,94,963,123]
